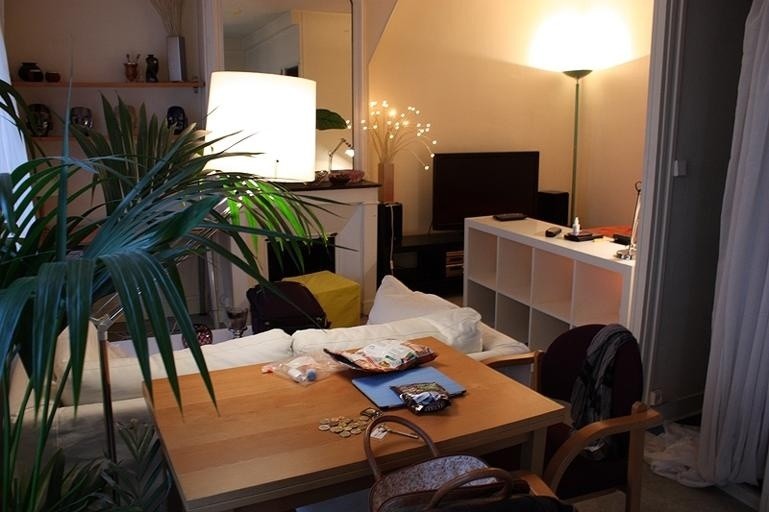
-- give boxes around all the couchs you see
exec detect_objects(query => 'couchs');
[6,274,530,498]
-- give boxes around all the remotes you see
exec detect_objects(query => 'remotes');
[544,226,562,237]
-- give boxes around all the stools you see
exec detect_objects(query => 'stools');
[280,270,361,327]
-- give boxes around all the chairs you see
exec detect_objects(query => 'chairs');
[477,348,660,512]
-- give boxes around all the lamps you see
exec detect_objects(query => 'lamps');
[527,8,638,228]
[199,69,317,185]
[345,99,437,202]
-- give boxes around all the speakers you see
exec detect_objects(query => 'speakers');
[378,201,403,245]
[538,190,569,226]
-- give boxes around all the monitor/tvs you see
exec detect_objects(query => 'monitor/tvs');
[431,150,540,231]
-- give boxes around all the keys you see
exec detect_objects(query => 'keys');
[360,406,419,443]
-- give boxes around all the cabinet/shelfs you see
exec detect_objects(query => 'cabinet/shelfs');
[462,211,636,362]
[393,233,464,296]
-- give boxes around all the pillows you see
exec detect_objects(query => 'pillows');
[292,306,484,356]
[54,319,118,374]
[56,329,292,404]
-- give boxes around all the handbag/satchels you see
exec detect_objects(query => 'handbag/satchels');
[366,414,530,511]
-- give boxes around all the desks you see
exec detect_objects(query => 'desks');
[212,176,383,322]
[140,334,565,512]
